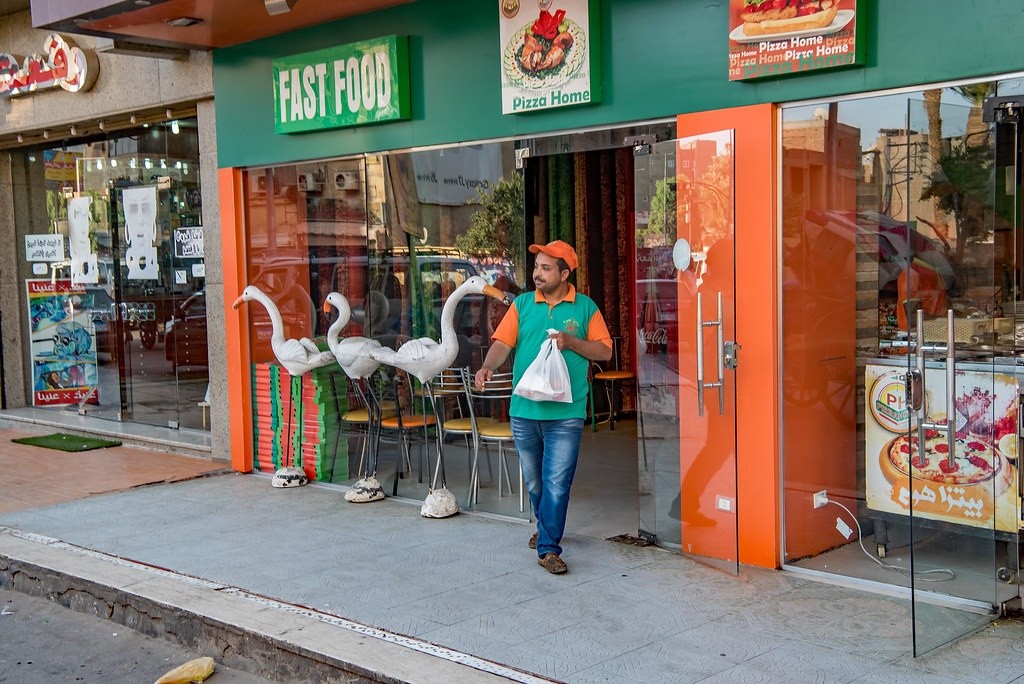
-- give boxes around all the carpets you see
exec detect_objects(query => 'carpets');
[11,432,123,452]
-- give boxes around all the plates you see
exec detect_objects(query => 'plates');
[729,9,855,43]
[504,15,586,91]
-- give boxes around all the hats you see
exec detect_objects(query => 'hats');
[529,240,579,272]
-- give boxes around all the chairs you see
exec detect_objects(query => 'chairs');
[327,367,446,497]
[426,367,514,505]
[459,367,525,513]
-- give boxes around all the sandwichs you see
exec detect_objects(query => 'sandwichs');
[741,0,840,36]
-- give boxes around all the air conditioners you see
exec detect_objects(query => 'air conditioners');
[296,173,323,192]
[252,175,266,193]
[334,172,359,191]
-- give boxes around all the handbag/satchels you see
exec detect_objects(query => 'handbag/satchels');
[513,338,573,403]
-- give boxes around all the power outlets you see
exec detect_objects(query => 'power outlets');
[813,490,828,509]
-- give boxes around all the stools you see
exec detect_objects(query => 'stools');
[596,371,635,431]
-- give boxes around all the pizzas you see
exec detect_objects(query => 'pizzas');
[891,431,999,483]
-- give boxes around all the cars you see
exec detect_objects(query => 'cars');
[636,279,695,350]
[164,284,304,375]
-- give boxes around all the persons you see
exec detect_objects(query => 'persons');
[896,222,964,333]
[473,241,613,576]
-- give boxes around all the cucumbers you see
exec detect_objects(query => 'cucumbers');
[504,18,585,88]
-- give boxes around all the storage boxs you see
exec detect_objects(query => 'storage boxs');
[251,335,351,485]
[923,316,1015,343]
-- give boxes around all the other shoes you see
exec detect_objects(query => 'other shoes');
[537,553,567,573]
[529,533,538,548]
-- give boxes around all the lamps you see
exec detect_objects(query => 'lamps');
[166,109,173,119]
[99,120,105,130]
[17,135,23,144]
[130,114,137,124]
[43,130,49,139]
[71,125,77,136]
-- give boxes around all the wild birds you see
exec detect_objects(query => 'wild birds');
[324,292,387,504]
[231,284,338,488]
[369,276,510,519]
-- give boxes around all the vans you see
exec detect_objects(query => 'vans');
[856,210,956,322]
[330,258,489,351]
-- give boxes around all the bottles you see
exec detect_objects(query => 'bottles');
[640,268,668,409]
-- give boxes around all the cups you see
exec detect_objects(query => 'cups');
[999,434,1018,458]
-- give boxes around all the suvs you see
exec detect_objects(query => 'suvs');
[71,286,120,362]
